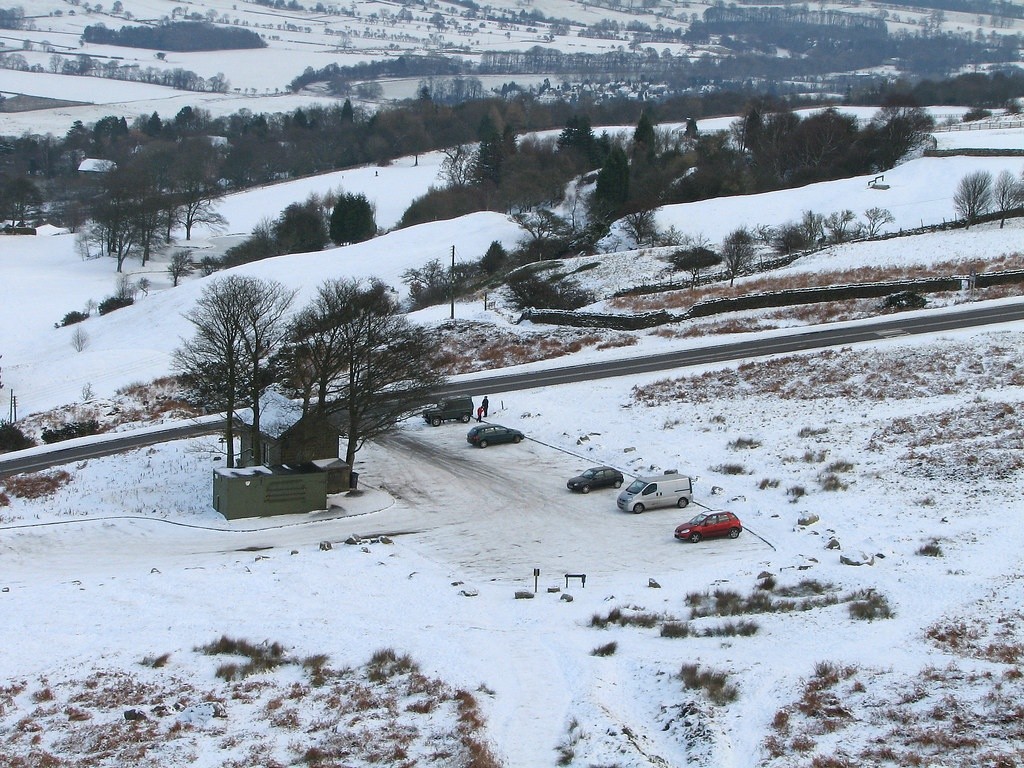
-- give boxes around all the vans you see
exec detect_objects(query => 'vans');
[617,474,693,514]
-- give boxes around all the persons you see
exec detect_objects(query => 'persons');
[476,406,484,422]
[482,396,488,417]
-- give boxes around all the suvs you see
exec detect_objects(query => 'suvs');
[674,510,742,544]
[567,467,624,494]
[422,396,473,427]
[466,424,524,448]
